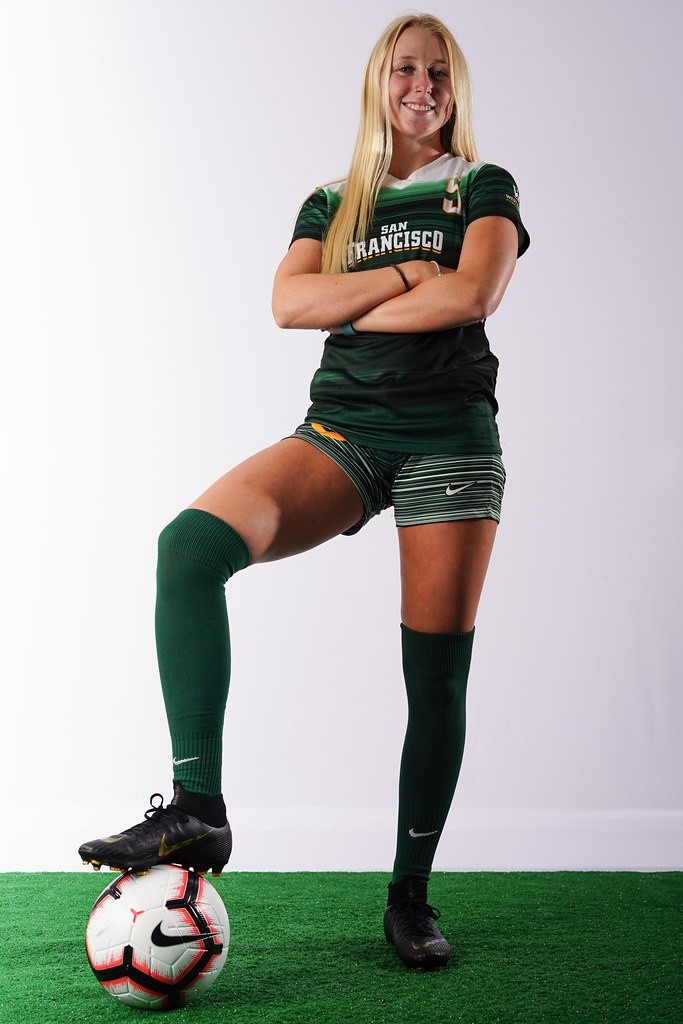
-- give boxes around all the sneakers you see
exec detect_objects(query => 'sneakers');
[382,881,451,970]
[77,782,232,877]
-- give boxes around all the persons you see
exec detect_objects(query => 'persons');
[74,12,528,972]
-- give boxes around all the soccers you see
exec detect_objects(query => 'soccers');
[85,862,232,1012]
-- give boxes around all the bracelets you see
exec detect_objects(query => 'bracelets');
[390,264,410,292]
[429,261,444,277]
